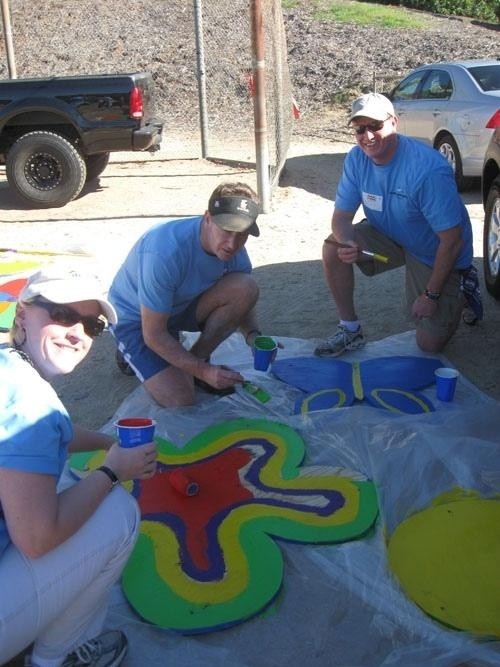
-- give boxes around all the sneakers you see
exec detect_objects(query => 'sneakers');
[24,629,129,667]
[192,375,235,396]
[462,266,483,326]
[115,347,137,375]
[315,322,366,359]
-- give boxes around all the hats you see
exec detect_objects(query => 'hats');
[350,92,395,122]
[209,194,261,237]
[20,255,120,326]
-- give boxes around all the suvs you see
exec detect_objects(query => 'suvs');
[478,117,500,293]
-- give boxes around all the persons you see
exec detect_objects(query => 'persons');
[314,92,485,360]
[0,260,160,667]
[106,181,286,408]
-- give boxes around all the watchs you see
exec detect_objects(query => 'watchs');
[422,288,442,302]
[96,465,121,494]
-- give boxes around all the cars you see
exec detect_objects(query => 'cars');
[380,59,499,186]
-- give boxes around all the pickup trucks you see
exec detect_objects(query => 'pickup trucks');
[0,71,166,208]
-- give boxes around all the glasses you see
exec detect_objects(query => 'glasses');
[352,116,391,134]
[31,298,105,338]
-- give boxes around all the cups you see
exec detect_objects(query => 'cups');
[433,367,459,401]
[115,416,156,448]
[253,334,278,370]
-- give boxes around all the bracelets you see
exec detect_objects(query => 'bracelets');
[243,329,263,346]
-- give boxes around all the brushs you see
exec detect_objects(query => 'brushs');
[326,239,389,264]
[241,381,271,404]
